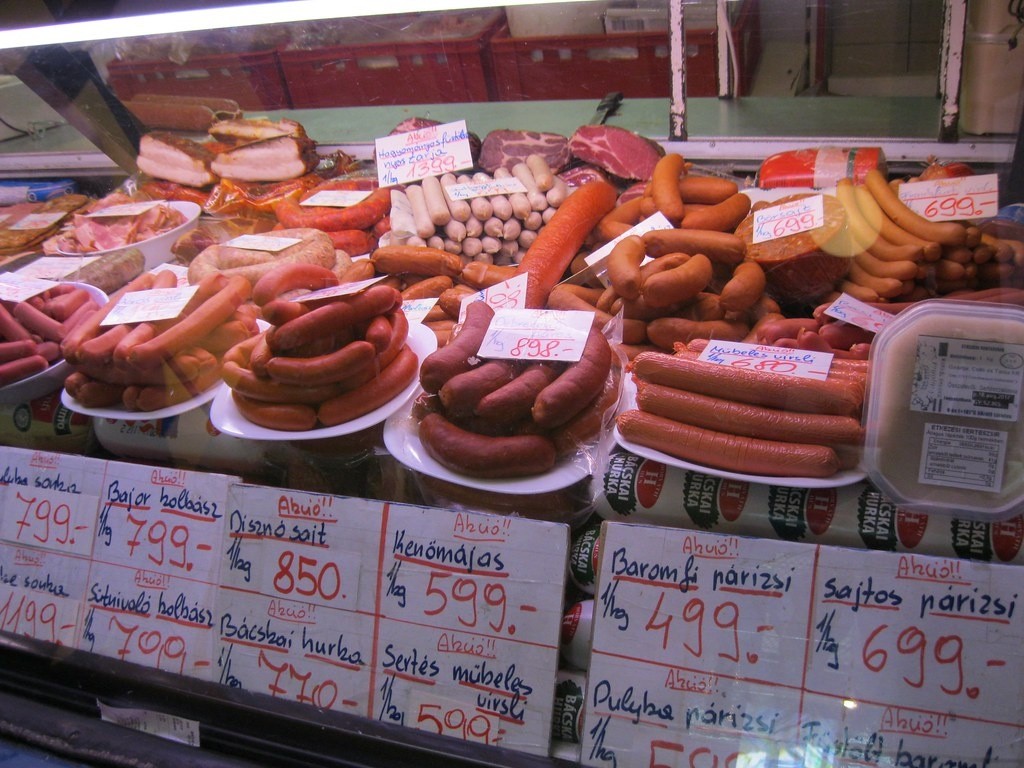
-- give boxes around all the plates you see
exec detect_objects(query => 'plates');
[210,323,437,440]
[612,413,868,487]
[382,372,635,495]
[60,318,273,420]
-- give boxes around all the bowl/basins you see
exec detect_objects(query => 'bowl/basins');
[857,299,1024,521]
[1,282,109,403]
[56,201,201,269]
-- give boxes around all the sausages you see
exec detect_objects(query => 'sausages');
[119,95,240,131]
[0,153,1024,479]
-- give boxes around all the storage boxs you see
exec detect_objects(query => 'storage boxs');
[105,46,291,112]
[489,0,760,100]
[276,8,507,110]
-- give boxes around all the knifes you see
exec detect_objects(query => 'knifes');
[588,91,621,124]
